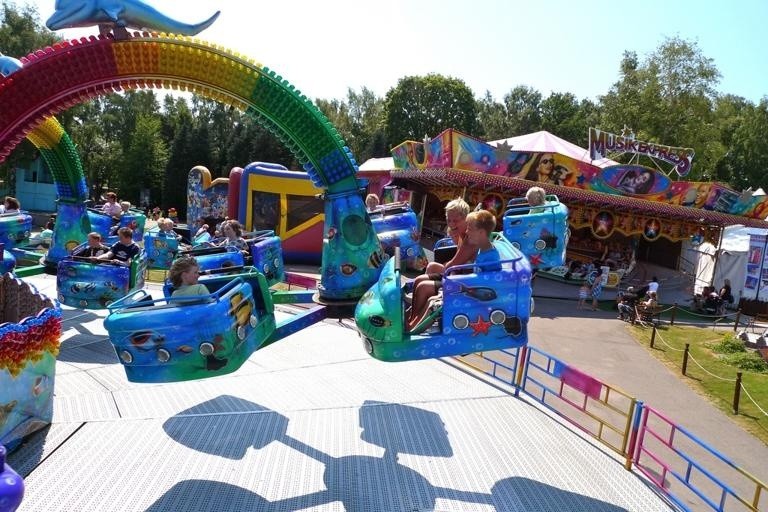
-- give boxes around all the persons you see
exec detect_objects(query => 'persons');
[365,194,385,218]
[682,184,712,213]
[524,153,565,187]
[615,170,654,196]
[46,218,56,231]
[389,186,548,332]
[566,237,659,322]
[0,196,21,216]
[85,192,249,304]
[699,279,731,315]
